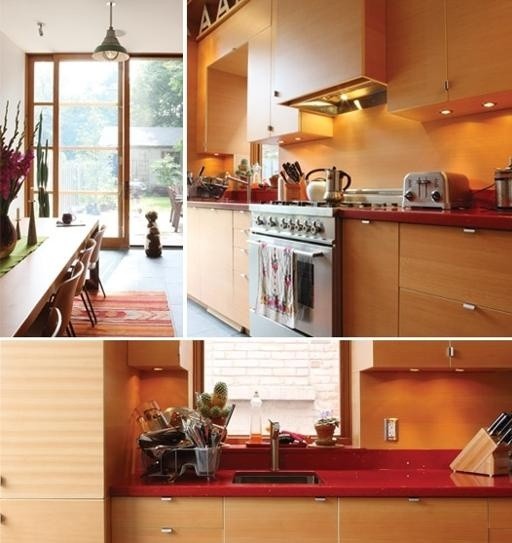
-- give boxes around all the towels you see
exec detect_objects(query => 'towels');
[256,242,298,329]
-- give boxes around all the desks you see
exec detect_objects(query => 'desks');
[1,216,100,336]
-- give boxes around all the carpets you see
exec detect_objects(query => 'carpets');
[64,288,177,337]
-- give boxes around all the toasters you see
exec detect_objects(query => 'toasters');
[402,170,470,210]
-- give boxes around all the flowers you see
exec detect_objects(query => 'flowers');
[316,408,341,427]
[1,99,43,218]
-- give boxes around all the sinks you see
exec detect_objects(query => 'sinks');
[233,470,328,485]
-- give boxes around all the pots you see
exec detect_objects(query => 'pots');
[493,158,512,209]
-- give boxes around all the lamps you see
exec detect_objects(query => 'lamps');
[91,1,131,62]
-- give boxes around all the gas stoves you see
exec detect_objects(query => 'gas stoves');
[247,199,340,246]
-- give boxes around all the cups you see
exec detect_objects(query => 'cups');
[194,448,217,473]
[62,213,78,225]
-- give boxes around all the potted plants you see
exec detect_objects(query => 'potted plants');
[195,380,236,444]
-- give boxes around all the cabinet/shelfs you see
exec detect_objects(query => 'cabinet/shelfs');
[126,339,191,373]
[185,205,234,323]
[384,0,512,122]
[349,341,511,374]
[233,209,252,331]
[0,340,140,543]
[341,216,398,337]
[108,495,224,543]
[225,497,488,541]
[195,0,333,156]
[488,497,512,543]
[399,222,511,336]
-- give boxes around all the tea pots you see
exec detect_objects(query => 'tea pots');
[324,165,351,207]
[305,167,330,201]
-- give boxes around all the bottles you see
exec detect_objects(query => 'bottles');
[277,171,286,200]
[247,160,262,204]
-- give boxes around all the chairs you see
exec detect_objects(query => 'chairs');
[167,185,183,230]
[31,223,108,339]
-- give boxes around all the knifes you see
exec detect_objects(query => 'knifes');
[279,160,302,183]
[488,408,512,444]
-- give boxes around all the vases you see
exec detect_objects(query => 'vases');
[315,420,337,445]
[1,214,19,259]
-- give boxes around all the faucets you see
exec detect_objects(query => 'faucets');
[226,170,252,203]
[267,416,280,471]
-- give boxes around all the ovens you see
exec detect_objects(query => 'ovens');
[246,232,342,336]
